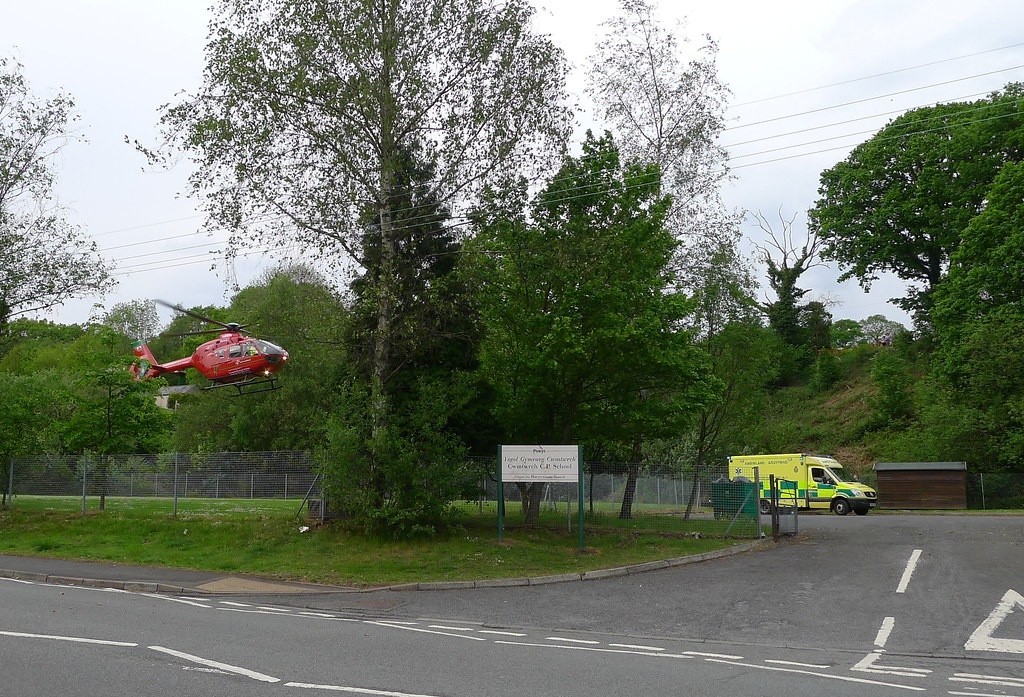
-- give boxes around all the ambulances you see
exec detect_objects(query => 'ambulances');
[727,452,878,516]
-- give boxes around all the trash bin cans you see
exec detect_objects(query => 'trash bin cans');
[711,477,763,517]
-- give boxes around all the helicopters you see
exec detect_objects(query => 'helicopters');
[127,298,291,397]
